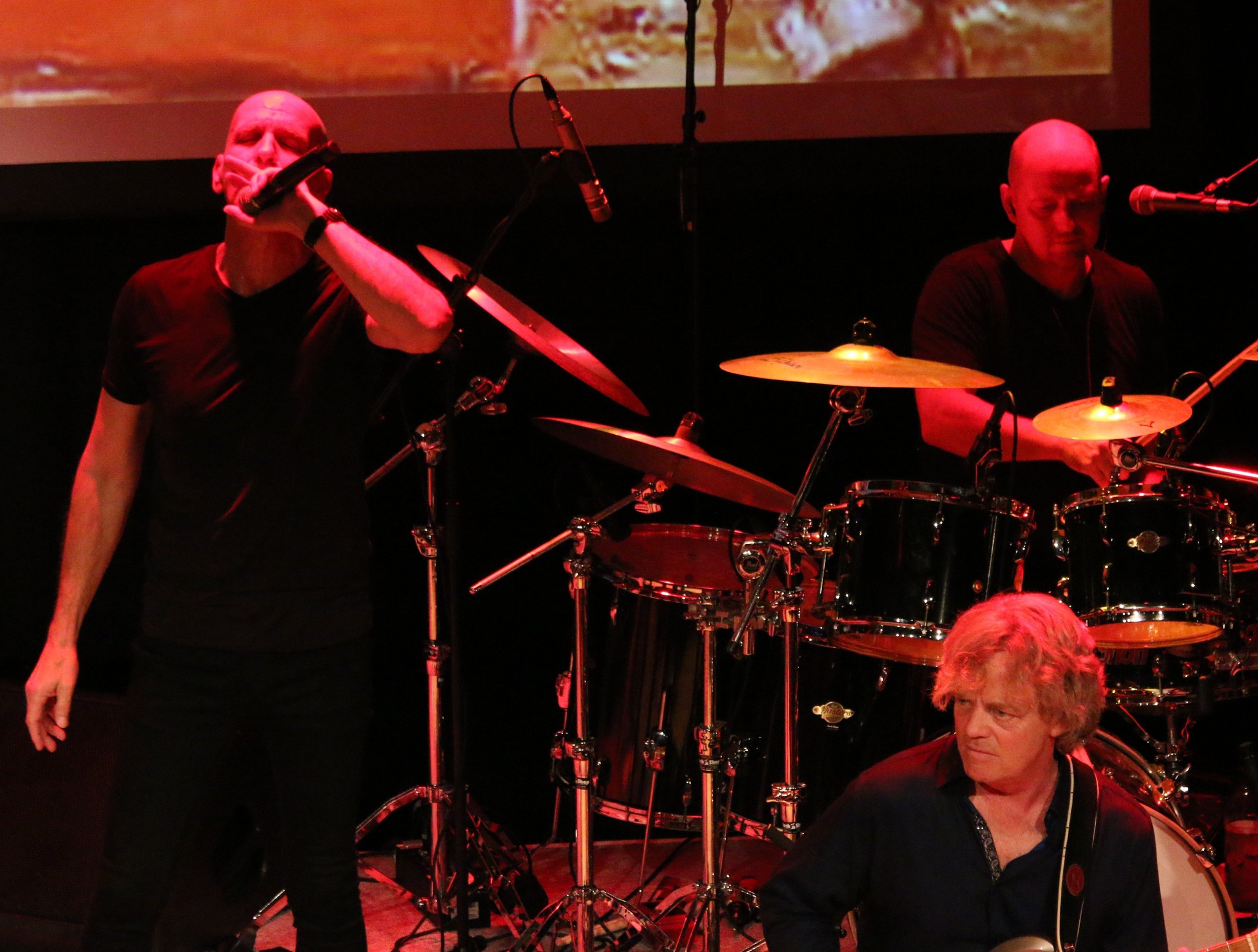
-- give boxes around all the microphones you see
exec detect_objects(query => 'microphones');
[1154,381,1182,457]
[967,391,1010,467]
[237,140,340,219]
[540,75,613,222]
[1129,186,1250,216]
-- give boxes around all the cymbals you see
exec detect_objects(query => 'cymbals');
[1031,392,1193,442]
[415,243,651,420]
[534,414,823,521]
[718,348,1006,393]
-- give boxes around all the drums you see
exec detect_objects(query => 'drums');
[830,478,1038,673]
[929,681,1189,832]
[711,574,853,851]
[1133,797,1240,952]
[1053,481,1229,652]
[579,522,785,834]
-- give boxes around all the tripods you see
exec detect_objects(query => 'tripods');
[231,275,761,951]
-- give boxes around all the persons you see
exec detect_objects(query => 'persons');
[759,592,1169,952]
[24,90,456,952]
[912,119,1167,489]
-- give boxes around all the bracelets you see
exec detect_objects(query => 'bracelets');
[302,207,345,251]
